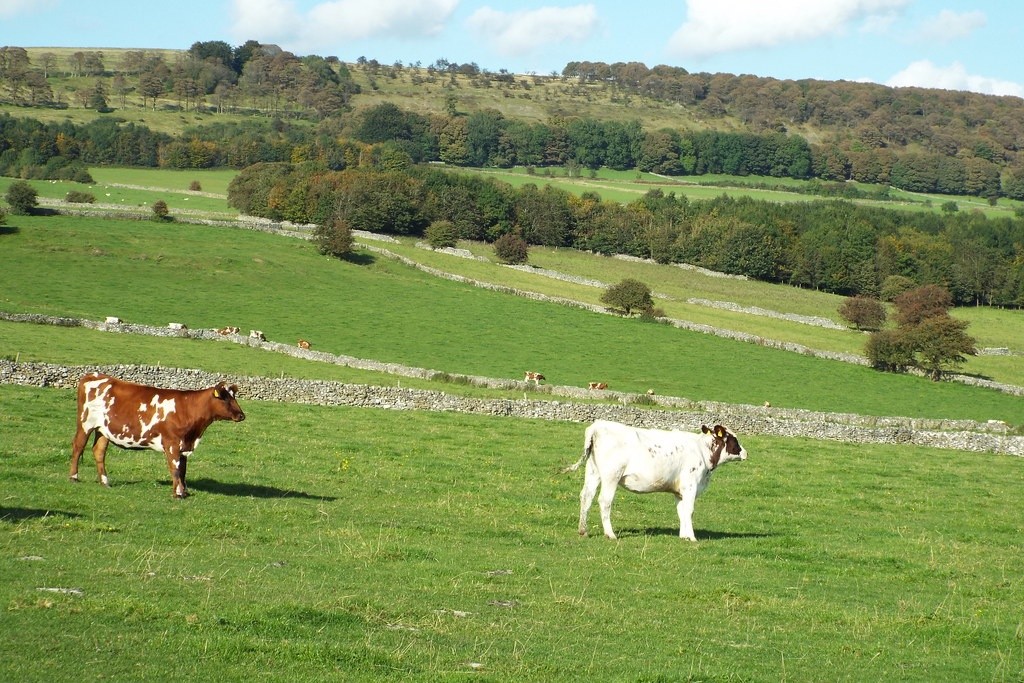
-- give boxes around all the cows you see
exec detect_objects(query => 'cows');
[298,339,310,349]
[70,372,246,498]
[558,419,750,541]
[524,369,546,385]
[589,382,608,390]
[167,322,187,329]
[105,317,123,324]
[250,330,266,341]
[210,326,240,335]
